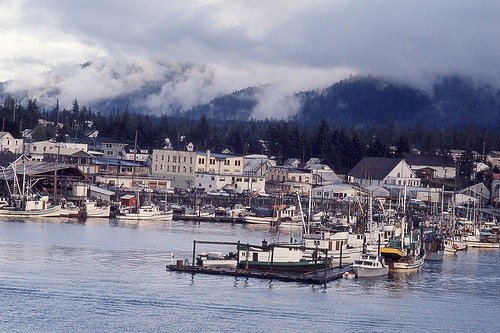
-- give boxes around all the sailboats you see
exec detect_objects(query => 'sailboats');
[291,168,499,271]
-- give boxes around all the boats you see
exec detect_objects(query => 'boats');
[349,254,390,278]
[56,195,112,218]
[242,211,279,224]
[115,205,175,222]
[0,162,61,218]
[194,251,237,267]
